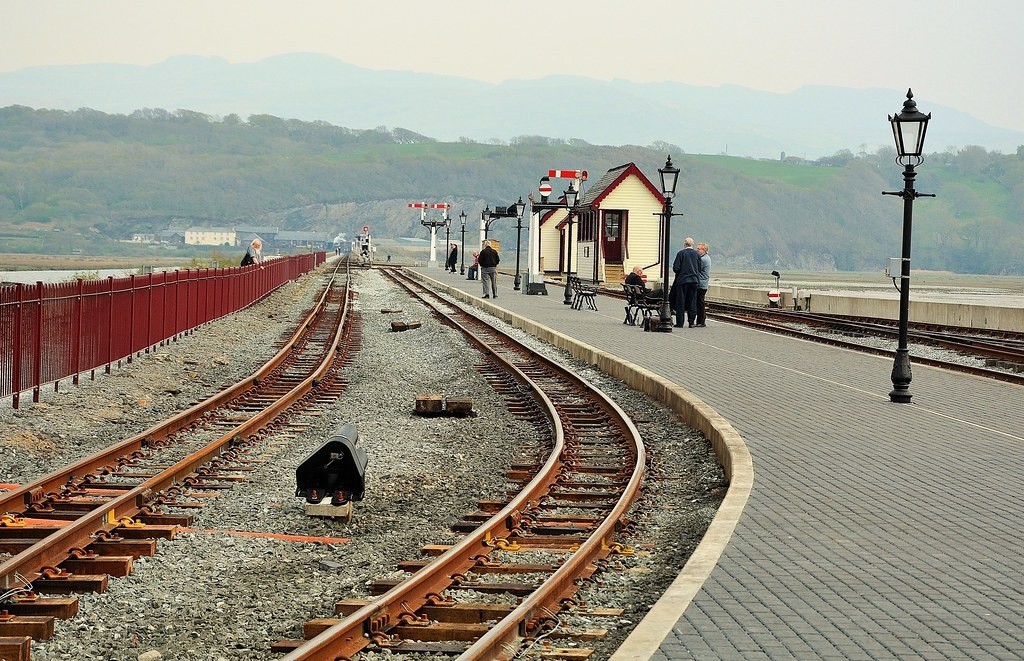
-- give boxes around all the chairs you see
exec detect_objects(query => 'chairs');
[473,266,478,279]
[570,277,598,311]
[619,283,664,327]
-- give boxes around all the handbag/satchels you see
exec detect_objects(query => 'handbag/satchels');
[241,254,254,266]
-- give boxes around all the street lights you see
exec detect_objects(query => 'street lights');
[444,215,452,269]
[562,181,579,308]
[481,205,492,242]
[512,196,527,291]
[651,154,685,329]
[882,86,937,402]
[459,210,469,276]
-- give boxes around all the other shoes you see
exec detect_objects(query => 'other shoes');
[493,295,500,299]
[689,323,697,328]
[482,294,490,299]
[673,323,684,328]
[696,322,707,327]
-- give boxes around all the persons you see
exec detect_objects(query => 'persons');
[336,248,341,255]
[673,238,711,328]
[478,240,500,299]
[448,243,458,272]
[241,238,264,270]
[466,251,478,280]
[387,255,391,262]
[624,266,664,304]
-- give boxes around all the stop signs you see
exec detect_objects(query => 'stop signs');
[539,184,552,196]
[769,290,781,303]
